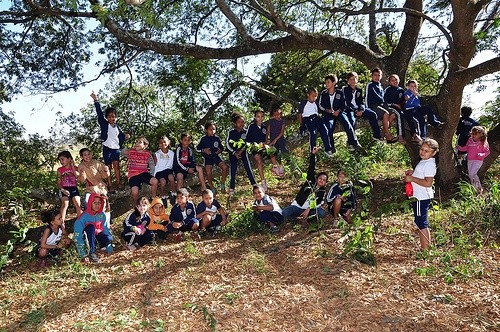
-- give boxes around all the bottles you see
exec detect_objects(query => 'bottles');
[405,172,413,196]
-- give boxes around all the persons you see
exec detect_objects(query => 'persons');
[383,74,422,144]
[57,151,83,230]
[366,68,399,144]
[252,184,284,232]
[327,170,356,227]
[457,125,490,190]
[78,148,110,209]
[123,122,227,202]
[283,147,328,228]
[74,194,112,261]
[456,106,478,160]
[122,188,227,251]
[299,87,331,154]
[37,210,70,267]
[318,74,362,152]
[406,139,439,250]
[403,80,446,139]
[90,92,130,194]
[342,72,384,148]
[226,108,291,194]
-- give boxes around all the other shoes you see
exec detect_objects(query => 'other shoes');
[229,189,234,195]
[355,144,365,151]
[270,225,280,232]
[373,134,383,142]
[411,135,422,141]
[108,185,115,193]
[117,183,125,190]
[348,145,355,152]
[88,253,99,262]
[261,179,268,192]
[332,149,336,156]
[332,219,339,227]
[324,150,335,158]
[279,166,285,177]
[272,165,280,176]
[438,121,447,127]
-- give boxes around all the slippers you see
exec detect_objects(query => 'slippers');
[387,137,399,143]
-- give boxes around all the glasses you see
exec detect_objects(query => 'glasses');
[92,201,102,204]
[421,149,433,153]
